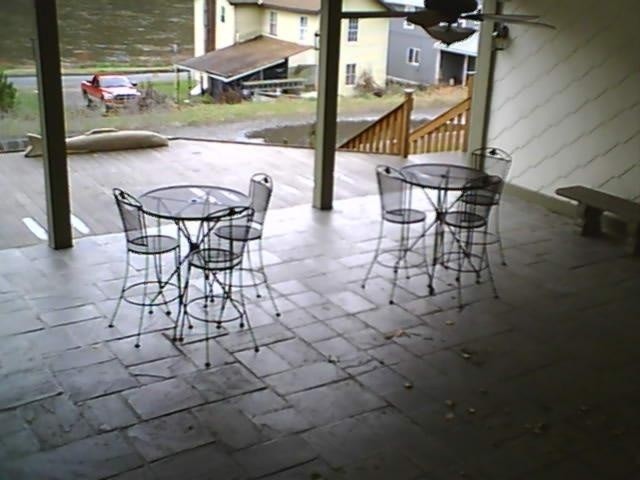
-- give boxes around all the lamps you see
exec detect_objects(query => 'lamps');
[492,26,509,50]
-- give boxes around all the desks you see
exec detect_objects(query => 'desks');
[394,164,488,288]
[138,185,253,342]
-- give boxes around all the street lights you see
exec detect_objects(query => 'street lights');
[168,43,181,102]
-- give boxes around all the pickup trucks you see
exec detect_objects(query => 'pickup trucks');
[79,73,143,117]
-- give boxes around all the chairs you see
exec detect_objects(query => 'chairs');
[173,207,260,368]
[362,165,434,304]
[427,176,504,311]
[108,188,194,348]
[446,147,512,283]
[204,172,281,329]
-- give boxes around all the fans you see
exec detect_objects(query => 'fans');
[338,0,556,45]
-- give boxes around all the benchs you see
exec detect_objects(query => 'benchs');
[556,186,640,256]
